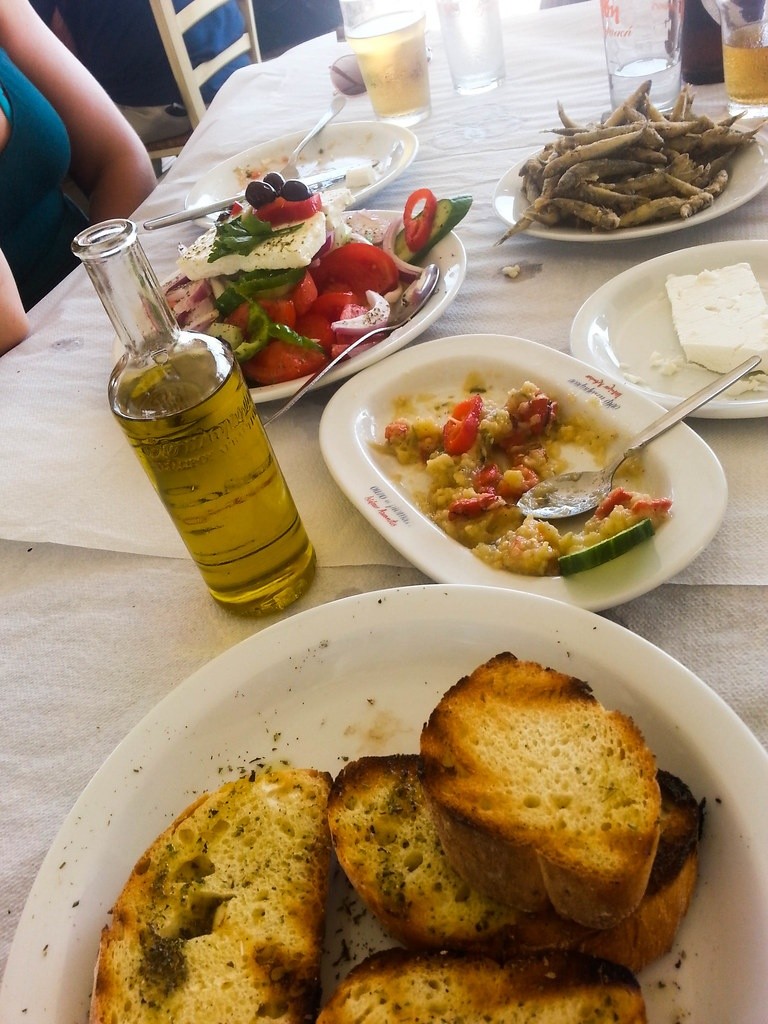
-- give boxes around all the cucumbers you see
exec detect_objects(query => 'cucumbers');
[558,517,653,575]
[395,195,473,264]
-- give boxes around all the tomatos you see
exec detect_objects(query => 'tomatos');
[225,241,399,384]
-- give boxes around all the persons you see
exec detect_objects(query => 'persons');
[0,0,158,357]
[29,0,254,177]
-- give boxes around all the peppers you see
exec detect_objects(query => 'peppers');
[403,188,438,253]
[213,268,325,360]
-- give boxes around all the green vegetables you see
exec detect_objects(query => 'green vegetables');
[208,213,304,261]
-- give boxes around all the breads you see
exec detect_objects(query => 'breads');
[89,651,700,1024]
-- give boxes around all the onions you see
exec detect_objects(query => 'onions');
[308,214,426,354]
[140,271,219,335]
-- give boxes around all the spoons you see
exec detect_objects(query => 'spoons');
[515,355,762,523]
[261,263,440,428]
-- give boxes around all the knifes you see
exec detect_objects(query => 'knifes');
[142,159,380,230]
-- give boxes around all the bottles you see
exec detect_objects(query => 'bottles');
[70,218,316,614]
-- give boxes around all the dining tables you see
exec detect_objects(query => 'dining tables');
[0,0,768,1024]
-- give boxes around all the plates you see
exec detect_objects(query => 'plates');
[493,121,768,244]
[111,209,468,402]
[568,240,768,420]
[1,584,767,1023]
[318,332,729,615]
[183,121,419,234]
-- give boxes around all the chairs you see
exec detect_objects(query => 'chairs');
[90,0,264,160]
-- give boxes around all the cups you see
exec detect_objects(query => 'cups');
[435,1,508,97]
[339,0,431,127]
[713,0,768,120]
[600,0,684,118]
